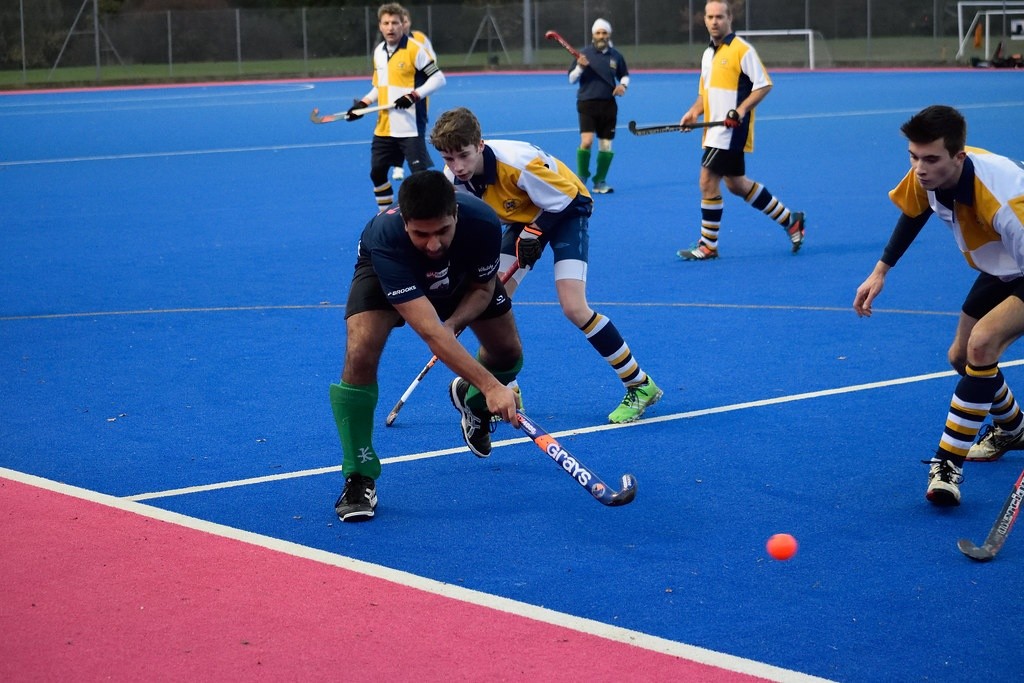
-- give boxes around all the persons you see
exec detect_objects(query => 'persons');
[330,171,522,519]
[346,3,446,212]
[677,0,804,257]
[854,105,1024,506]
[569,19,629,192]
[430,107,664,424]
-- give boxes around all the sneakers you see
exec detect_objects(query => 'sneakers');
[609,375,663,424]
[965,411,1024,462]
[489,388,525,423]
[335,474,378,521]
[592,180,613,194]
[784,210,805,254]
[449,376,497,458]
[921,456,964,506]
[677,238,718,260]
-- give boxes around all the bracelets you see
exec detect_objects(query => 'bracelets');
[623,84,627,88]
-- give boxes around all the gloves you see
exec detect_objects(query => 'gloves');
[515,223,542,269]
[345,101,368,122]
[394,93,415,110]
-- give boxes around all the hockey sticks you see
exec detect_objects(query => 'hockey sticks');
[628,121,742,137]
[514,408,639,507]
[545,31,615,90]
[957,470,1024,562]
[310,102,397,125]
[386,258,521,427]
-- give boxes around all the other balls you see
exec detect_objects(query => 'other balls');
[765,533,799,562]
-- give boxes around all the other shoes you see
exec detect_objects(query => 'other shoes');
[392,167,405,180]
